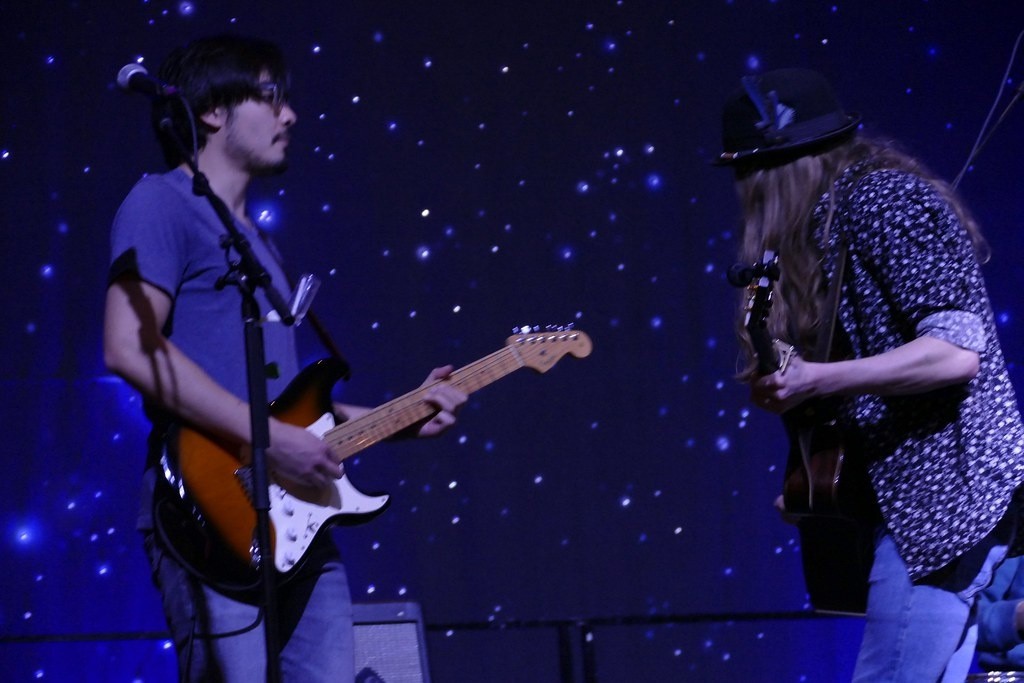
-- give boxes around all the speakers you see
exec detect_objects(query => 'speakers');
[349,601,432,683]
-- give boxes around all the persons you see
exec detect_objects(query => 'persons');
[715,68,1024,683]
[104,34,467,683]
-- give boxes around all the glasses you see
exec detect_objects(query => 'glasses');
[259,78,289,113]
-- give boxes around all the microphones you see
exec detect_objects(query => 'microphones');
[116,62,177,99]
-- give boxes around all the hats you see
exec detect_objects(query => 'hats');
[706,66,862,166]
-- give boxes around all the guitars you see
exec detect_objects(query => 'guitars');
[741,253,874,615]
[145,320,593,609]
